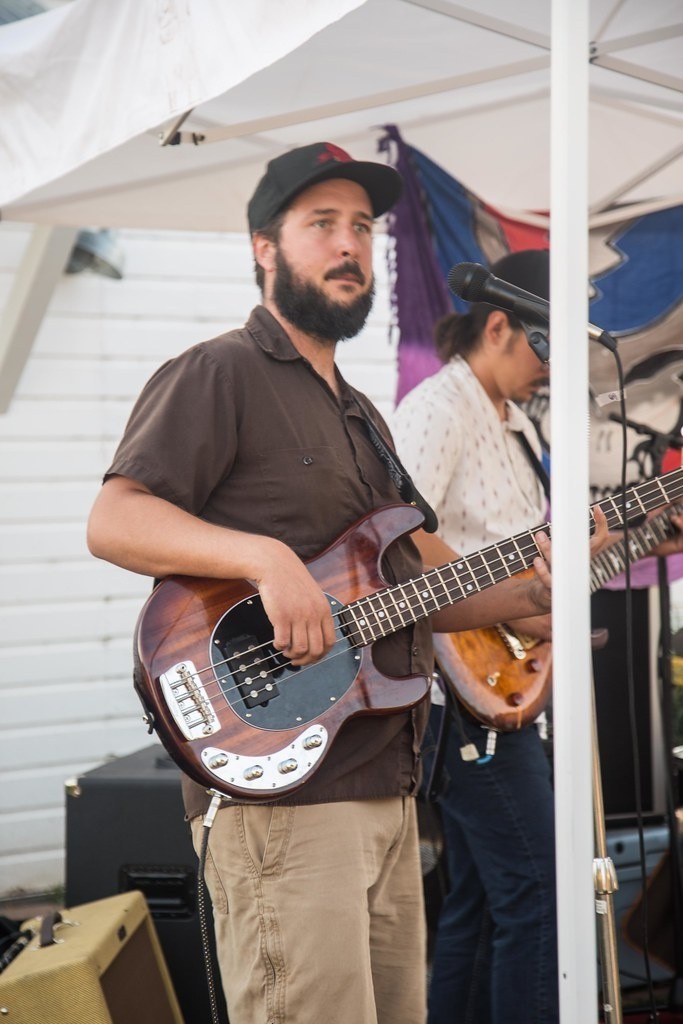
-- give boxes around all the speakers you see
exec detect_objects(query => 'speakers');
[65,745,228,1023]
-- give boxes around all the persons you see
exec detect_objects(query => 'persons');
[392,247,683,1023]
[85,141,612,1024]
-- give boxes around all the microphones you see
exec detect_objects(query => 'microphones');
[449,261,618,353]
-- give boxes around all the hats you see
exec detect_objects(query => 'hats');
[248,142,406,240]
[483,247,552,323]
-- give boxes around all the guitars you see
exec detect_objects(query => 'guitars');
[131,469,683,801]
[427,497,683,727]
